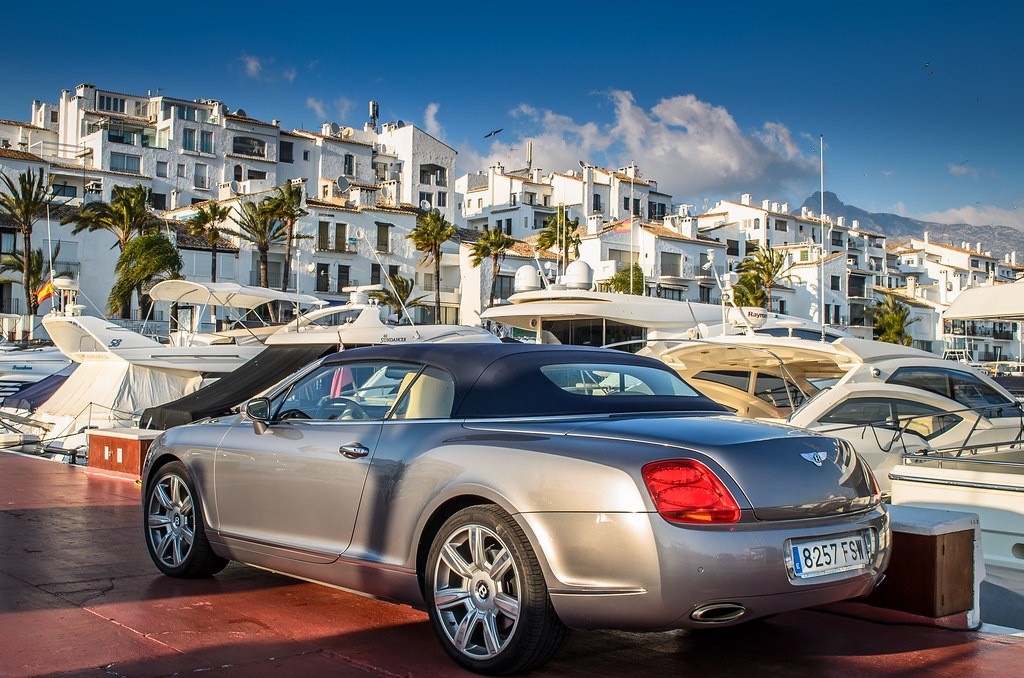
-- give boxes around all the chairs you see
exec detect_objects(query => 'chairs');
[385,370,454,418]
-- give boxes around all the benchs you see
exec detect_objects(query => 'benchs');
[844,505,985,627]
[83,428,165,482]
[885,414,934,437]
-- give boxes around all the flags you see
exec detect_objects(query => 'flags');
[34,279,53,304]
[329,344,353,400]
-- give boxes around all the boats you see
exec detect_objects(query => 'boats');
[0,133,1024,599]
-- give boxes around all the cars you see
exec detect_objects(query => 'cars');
[139,336,892,678]
